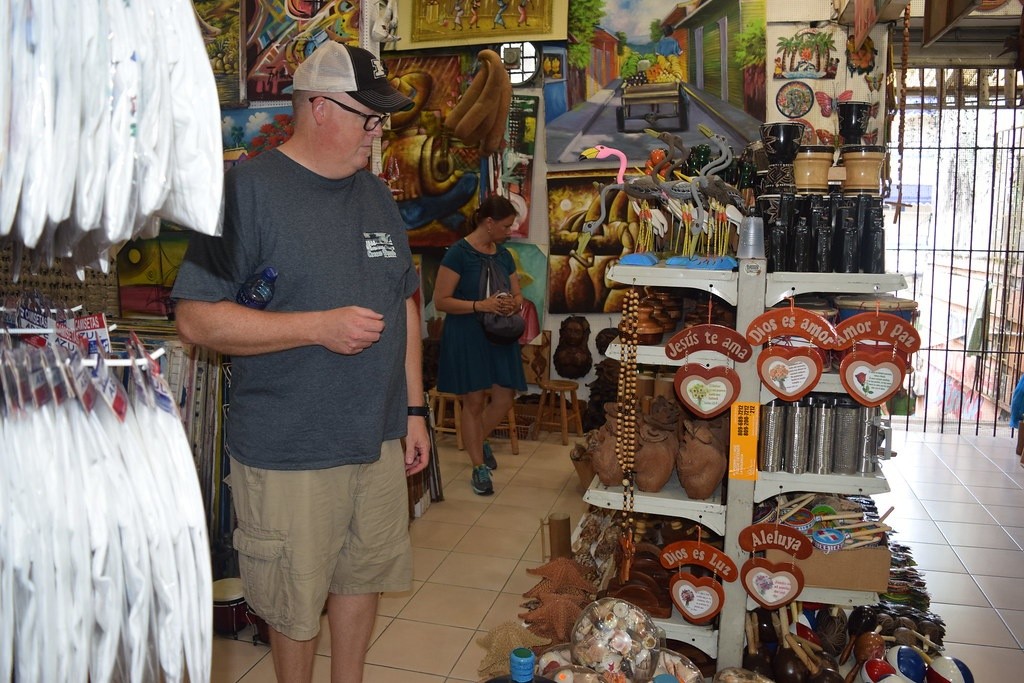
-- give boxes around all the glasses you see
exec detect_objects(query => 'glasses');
[309,95,389,131]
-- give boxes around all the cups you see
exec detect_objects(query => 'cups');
[791,145,836,196]
[840,143,885,199]
[735,216,765,259]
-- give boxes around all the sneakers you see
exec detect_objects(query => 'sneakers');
[482,438,497,469]
[471,464,495,496]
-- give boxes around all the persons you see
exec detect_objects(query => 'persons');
[168,40,432,682]
[433,196,528,496]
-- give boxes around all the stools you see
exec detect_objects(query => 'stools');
[427,379,585,455]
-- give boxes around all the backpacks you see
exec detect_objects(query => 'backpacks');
[457,244,526,344]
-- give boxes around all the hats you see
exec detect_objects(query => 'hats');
[293,40,415,114]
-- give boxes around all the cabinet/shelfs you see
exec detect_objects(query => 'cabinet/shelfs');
[581,215,907,683]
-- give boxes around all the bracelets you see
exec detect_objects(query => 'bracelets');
[408,406,430,419]
[473,300,477,313]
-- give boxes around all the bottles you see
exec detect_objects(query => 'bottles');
[483,647,559,683]
[759,395,880,475]
[235,267,278,310]
